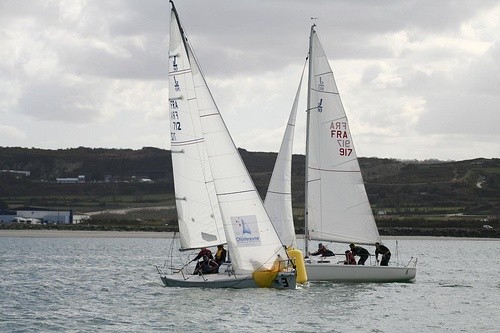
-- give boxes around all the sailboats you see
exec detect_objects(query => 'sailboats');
[264,24,418,283]
[153,0,297,290]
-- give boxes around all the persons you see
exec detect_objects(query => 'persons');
[308,243,335,256]
[375,243,391,266]
[192,245,226,275]
[349,243,370,265]
[344,250,356,265]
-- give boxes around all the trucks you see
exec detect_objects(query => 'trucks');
[12,216,42,224]
